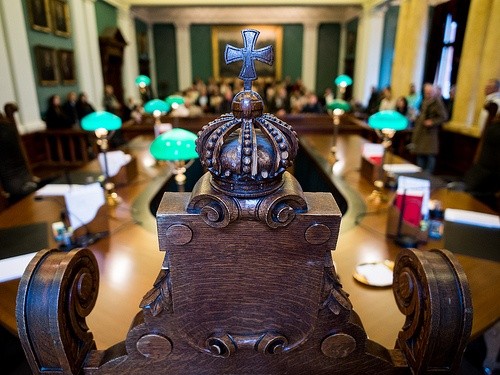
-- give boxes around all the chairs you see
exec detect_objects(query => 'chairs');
[16,29,473,375]
[0,103,33,201]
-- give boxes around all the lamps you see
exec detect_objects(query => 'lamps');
[365,110,409,204]
[149,128,200,193]
[81,112,122,206]
[144,99,168,135]
[325,99,352,161]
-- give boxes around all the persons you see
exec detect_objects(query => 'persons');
[44,92,95,161]
[105,85,131,123]
[365,78,500,190]
[184,74,334,116]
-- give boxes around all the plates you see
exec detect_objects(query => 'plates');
[353,260,395,286]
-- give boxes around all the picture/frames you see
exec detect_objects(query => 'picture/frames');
[210,25,284,84]
[57,49,76,85]
[27,0,53,33]
[34,46,58,87]
[51,0,71,37]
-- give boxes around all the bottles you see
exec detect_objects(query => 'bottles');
[382,175,398,208]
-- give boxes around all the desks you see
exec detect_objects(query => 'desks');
[0,133,500,375]
